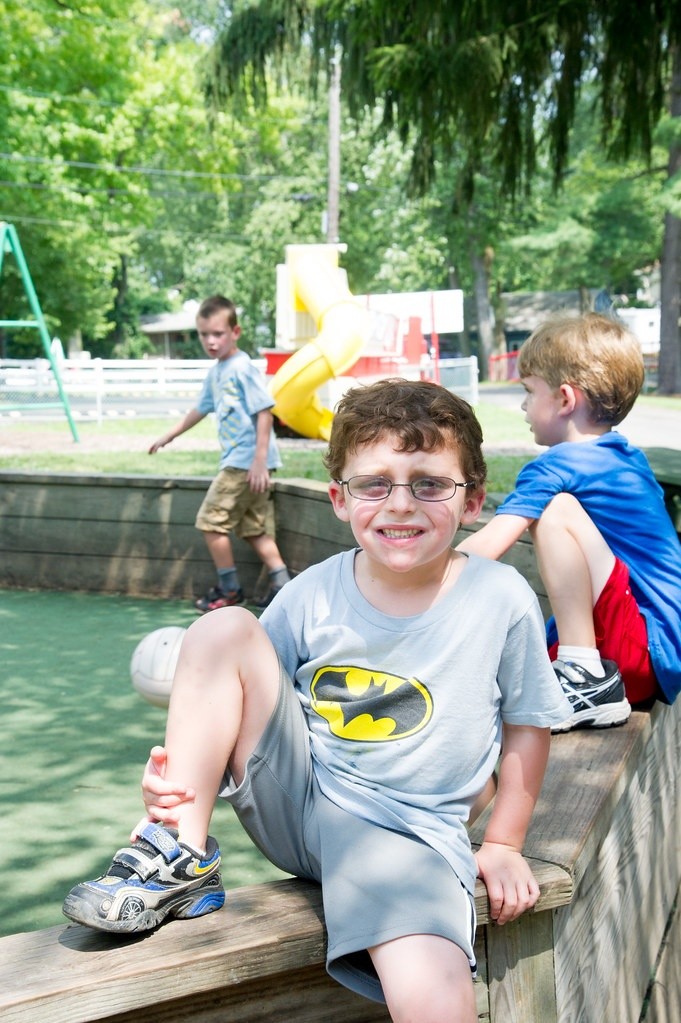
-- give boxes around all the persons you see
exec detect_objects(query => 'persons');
[148,294,293,612]
[66,376,574,1023]
[452,312,681,736]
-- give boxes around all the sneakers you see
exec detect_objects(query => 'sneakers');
[197,589,247,613]
[549,659,631,734]
[63,823,224,933]
[257,596,273,610]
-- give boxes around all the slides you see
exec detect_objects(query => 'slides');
[268,243,375,440]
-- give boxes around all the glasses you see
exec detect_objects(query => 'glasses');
[333,475,474,502]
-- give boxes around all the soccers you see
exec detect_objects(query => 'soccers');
[129,626,188,710]
